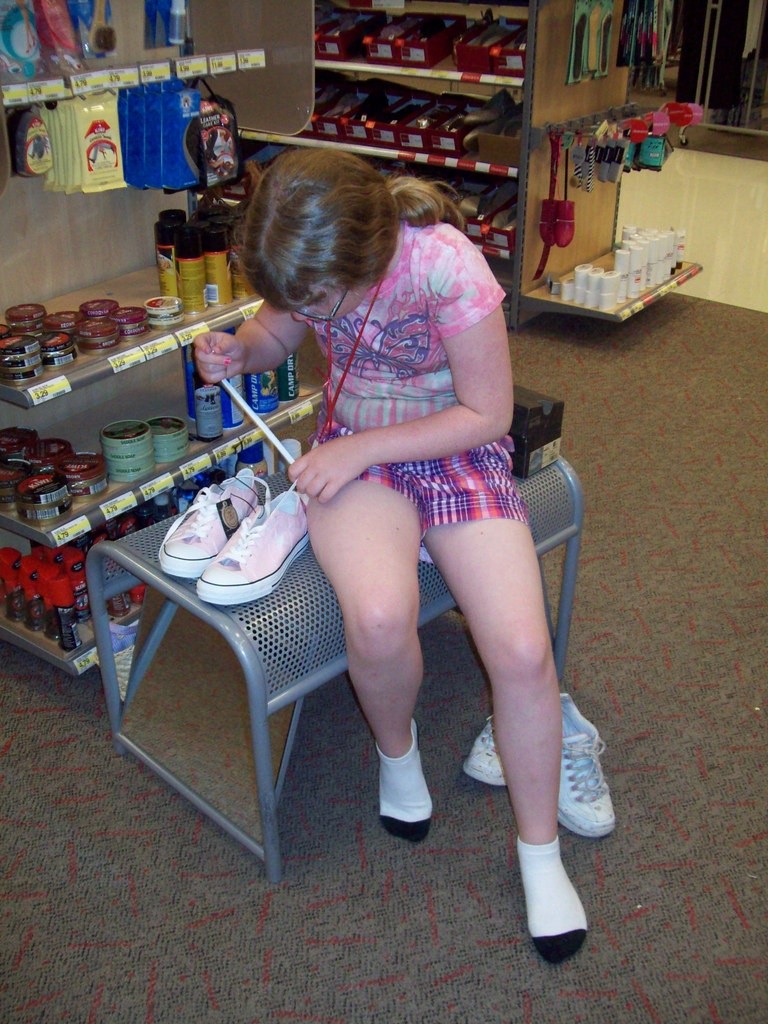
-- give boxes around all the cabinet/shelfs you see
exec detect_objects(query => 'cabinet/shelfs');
[0,265,326,676]
[234,0,704,332]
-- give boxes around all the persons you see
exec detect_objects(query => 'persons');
[195,147,589,966]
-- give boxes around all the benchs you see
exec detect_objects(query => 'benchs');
[83,454,583,884]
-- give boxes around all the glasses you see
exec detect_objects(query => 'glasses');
[294,280,351,322]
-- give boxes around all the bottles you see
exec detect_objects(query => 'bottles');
[182,337,300,442]
[613,225,686,303]
[1,504,180,652]
[154,208,258,316]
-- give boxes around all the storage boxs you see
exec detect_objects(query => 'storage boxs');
[368,157,517,257]
[506,385,564,479]
[312,6,526,77]
[296,76,524,167]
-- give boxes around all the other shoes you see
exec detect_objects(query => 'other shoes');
[314,2,530,246]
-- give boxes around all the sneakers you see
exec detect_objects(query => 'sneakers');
[195,475,308,605]
[551,693,616,837]
[463,714,509,791]
[158,469,271,580]
